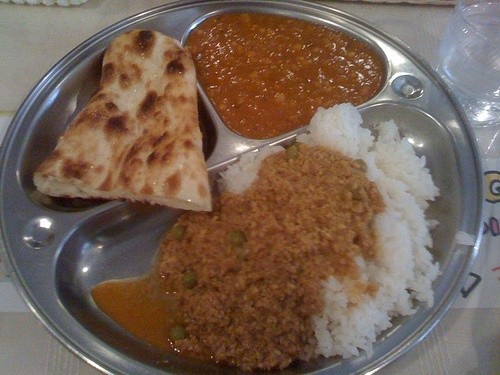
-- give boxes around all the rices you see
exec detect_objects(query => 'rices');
[218,103,443,359]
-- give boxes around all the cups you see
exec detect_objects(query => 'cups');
[441,1,500,129]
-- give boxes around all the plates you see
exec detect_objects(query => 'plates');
[0,1,482,375]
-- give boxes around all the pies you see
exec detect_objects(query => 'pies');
[33,29,213,210]
